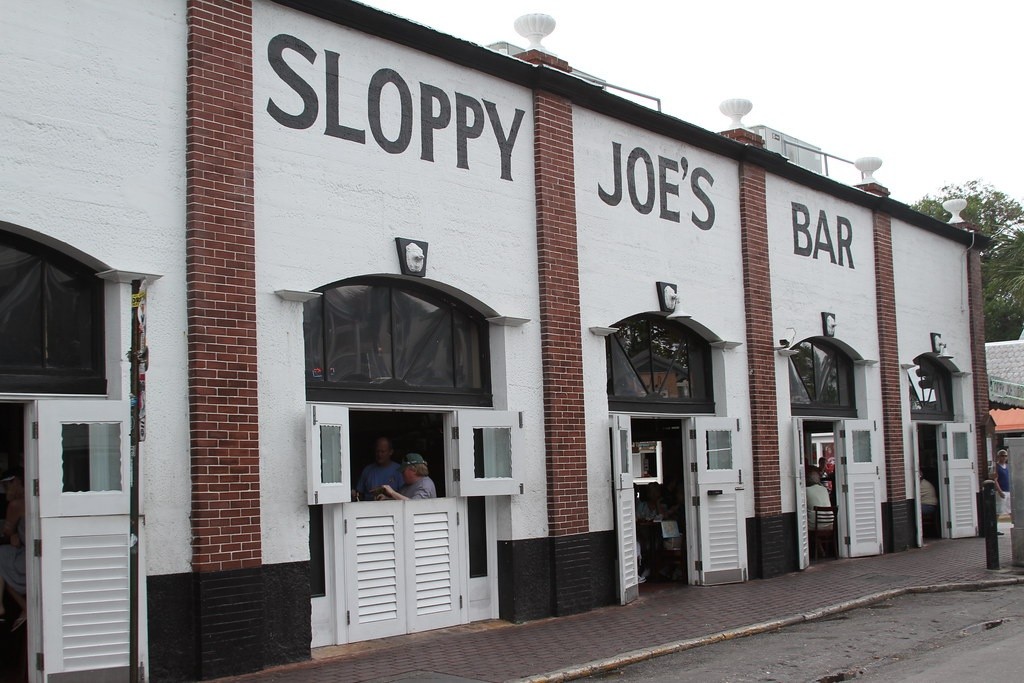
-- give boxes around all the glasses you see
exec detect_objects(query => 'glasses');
[1000,455,1008,458]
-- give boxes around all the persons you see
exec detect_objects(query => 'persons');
[0,451,27,633]
[920,471,939,513]
[351,437,404,502]
[806,457,835,559]
[635,474,684,583]
[993,449,1009,534]
[369,453,437,502]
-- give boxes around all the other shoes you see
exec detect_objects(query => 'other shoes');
[997,531,1004,535]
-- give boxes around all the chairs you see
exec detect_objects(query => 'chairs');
[922,499,940,537]
[813,506,839,560]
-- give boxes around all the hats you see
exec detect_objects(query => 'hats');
[396,453,426,473]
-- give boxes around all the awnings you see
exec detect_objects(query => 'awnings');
[988,376,1024,434]
